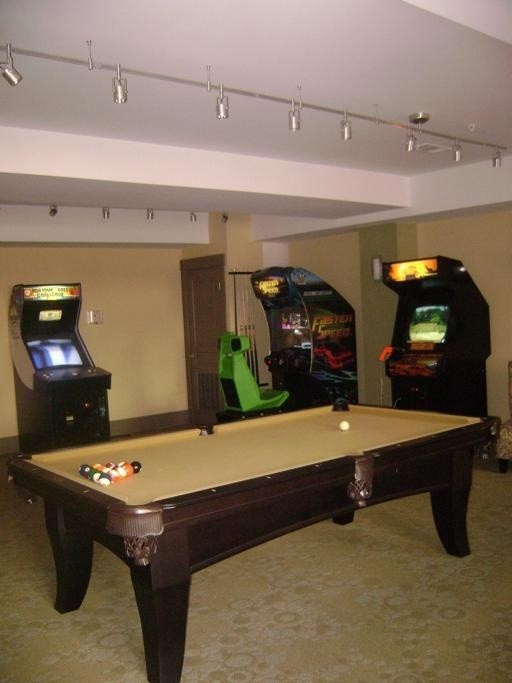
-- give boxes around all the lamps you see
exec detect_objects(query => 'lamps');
[0,42,508,167]
[49,204,229,225]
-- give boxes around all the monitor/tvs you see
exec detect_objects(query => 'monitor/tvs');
[275,308,311,350]
[406,306,451,345]
[24,336,84,371]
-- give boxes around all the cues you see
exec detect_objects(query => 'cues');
[234,267,260,388]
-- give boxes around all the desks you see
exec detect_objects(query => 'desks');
[7,397,501,683]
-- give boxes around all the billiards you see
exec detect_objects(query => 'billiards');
[78,461,143,485]
[340,421,349,431]
